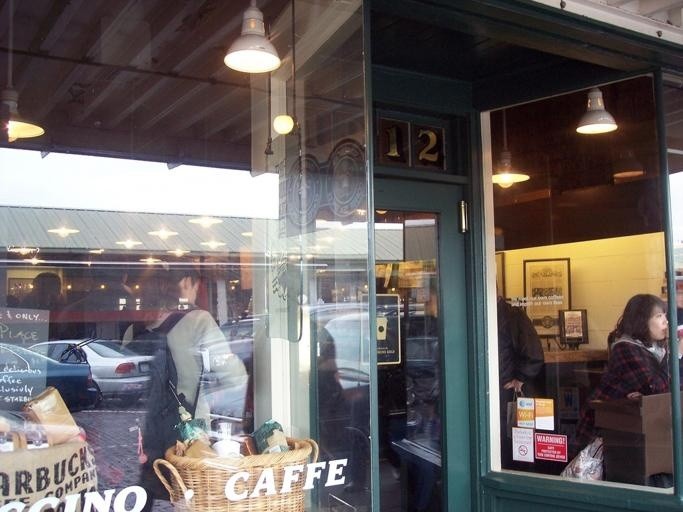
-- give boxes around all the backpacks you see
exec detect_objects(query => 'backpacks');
[104,309,199,500]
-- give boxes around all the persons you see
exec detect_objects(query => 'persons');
[495,280,544,464]
[574,292,682,491]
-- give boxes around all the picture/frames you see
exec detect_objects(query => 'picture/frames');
[496,251,505,303]
[523,257,571,338]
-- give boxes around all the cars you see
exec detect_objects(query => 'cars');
[0,303,157,440]
[222,300,440,452]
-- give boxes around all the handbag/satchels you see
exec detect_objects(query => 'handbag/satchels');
[506,391,525,441]
[0,385,100,512]
[560,436,604,482]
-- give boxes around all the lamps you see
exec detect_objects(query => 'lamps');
[0,0,46,143]
[222,0,281,73]
[575,88,618,133]
[492,108,530,188]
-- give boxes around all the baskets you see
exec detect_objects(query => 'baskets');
[152,436,319,512]
